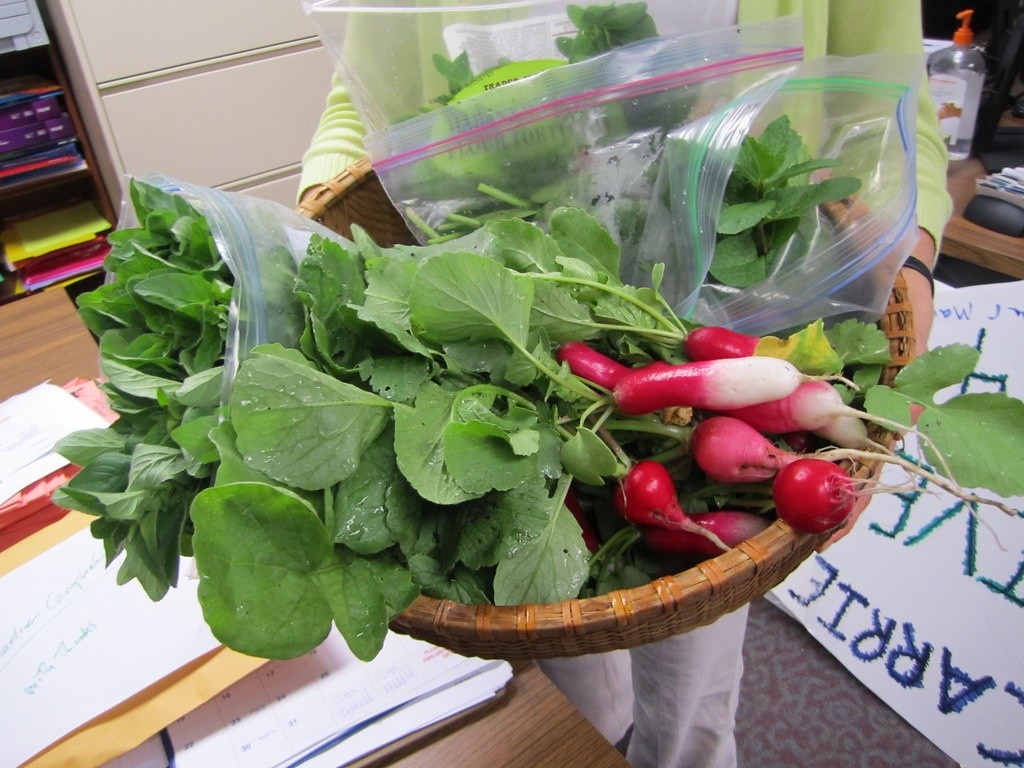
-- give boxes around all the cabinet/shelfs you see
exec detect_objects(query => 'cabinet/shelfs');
[0,0,338,299]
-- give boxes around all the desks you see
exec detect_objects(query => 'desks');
[943,156,1023,283]
[0,287,641,768]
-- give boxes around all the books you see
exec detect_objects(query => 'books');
[0,75,82,187]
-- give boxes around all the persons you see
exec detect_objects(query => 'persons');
[296,0,953,768]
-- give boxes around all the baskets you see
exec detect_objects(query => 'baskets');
[262,152,917,660]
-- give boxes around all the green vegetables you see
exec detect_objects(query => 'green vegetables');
[49,179,340,604]
[391,2,864,294]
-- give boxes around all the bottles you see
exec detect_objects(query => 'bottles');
[927,10,987,160]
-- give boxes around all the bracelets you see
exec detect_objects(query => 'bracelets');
[903,255,936,298]
